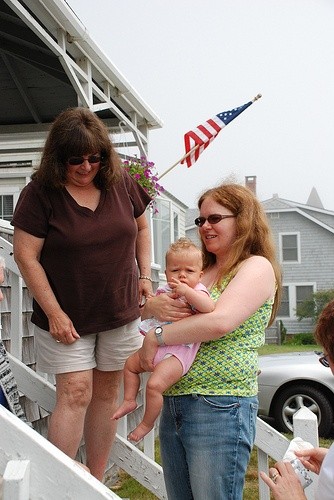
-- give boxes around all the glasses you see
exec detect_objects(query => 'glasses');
[319,354,332,367]
[195,214,237,227]
[67,156,104,165]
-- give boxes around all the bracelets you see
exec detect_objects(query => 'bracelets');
[139,276,151,281]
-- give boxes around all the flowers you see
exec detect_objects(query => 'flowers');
[123,154,164,215]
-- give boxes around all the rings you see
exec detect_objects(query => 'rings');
[272,473,278,480]
[56,340,61,343]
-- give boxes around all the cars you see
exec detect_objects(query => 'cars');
[257,349,334,439]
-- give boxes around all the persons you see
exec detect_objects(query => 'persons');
[141,186,284,500]
[260,298,334,500]
[109,234,214,442]
[0,256,34,430]
[10,108,152,482]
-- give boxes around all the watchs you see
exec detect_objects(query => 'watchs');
[154,327,163,344]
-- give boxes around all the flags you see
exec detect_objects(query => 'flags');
[181,100,253,168]
